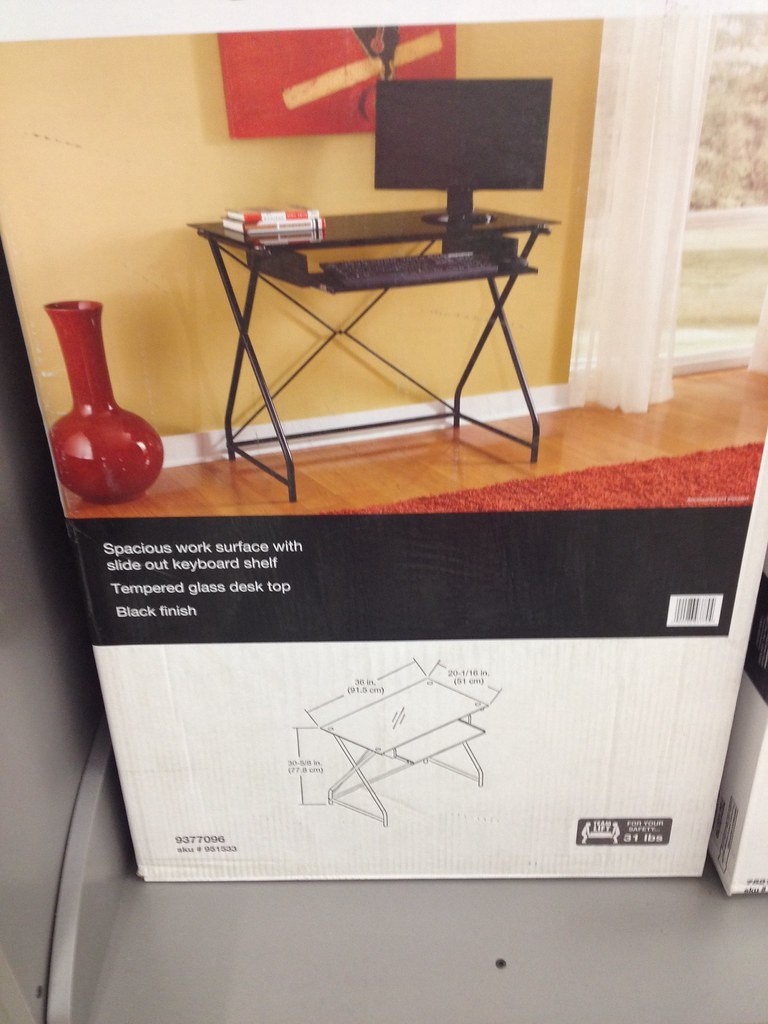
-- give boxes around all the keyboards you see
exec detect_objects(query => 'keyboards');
[319,249,499,290]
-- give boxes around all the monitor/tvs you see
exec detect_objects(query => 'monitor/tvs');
[374,78,553,225]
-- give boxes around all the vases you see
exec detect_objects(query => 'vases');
[44,298,164,504]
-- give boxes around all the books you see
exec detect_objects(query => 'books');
[220,205,326,236]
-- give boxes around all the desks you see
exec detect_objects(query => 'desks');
[188,206,564,504]
